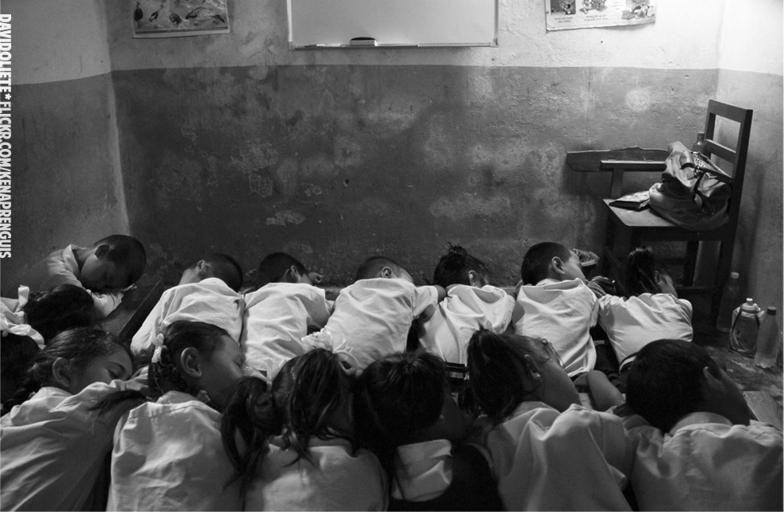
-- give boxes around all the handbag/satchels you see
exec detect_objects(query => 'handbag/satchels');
[649,141,731,232]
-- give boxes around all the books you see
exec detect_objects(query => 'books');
[608,190,650,212]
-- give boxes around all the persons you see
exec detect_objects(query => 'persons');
[1,234,699,401]
[0,324,149,512]
[573,338,782,510]
[220,347,389,512]
[348,351,508,511]
[84,319,269,512]
[457,328,634,512]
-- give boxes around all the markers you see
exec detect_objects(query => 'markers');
[304,44,323,47]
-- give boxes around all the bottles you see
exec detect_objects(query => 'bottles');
[715,271,742,332]
[729,295,763,354]
[691,130,705,155]
[752,304,780,370]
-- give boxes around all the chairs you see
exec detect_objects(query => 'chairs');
[603,99,754,330]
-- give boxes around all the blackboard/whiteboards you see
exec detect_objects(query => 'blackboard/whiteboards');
[286,0,500,51]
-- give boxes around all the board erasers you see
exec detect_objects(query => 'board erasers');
[351,37,376,46]
